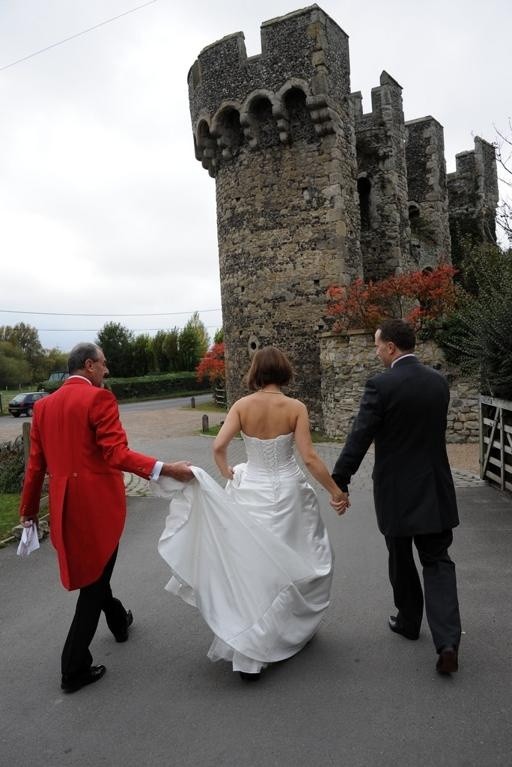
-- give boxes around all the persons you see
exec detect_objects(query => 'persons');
[17,339,198,692]
[211,345,350,682]
[330,319,464,673]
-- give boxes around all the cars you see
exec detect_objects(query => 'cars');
[9,391,51,416]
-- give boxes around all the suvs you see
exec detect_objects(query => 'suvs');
[38,371,69,393]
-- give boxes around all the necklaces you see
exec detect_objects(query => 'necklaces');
[258,388,283,394]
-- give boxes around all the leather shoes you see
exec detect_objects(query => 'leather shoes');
[435,643,459,674]
[388,615,421,641]
[113,608,134,643]
[62,662,107,694]
